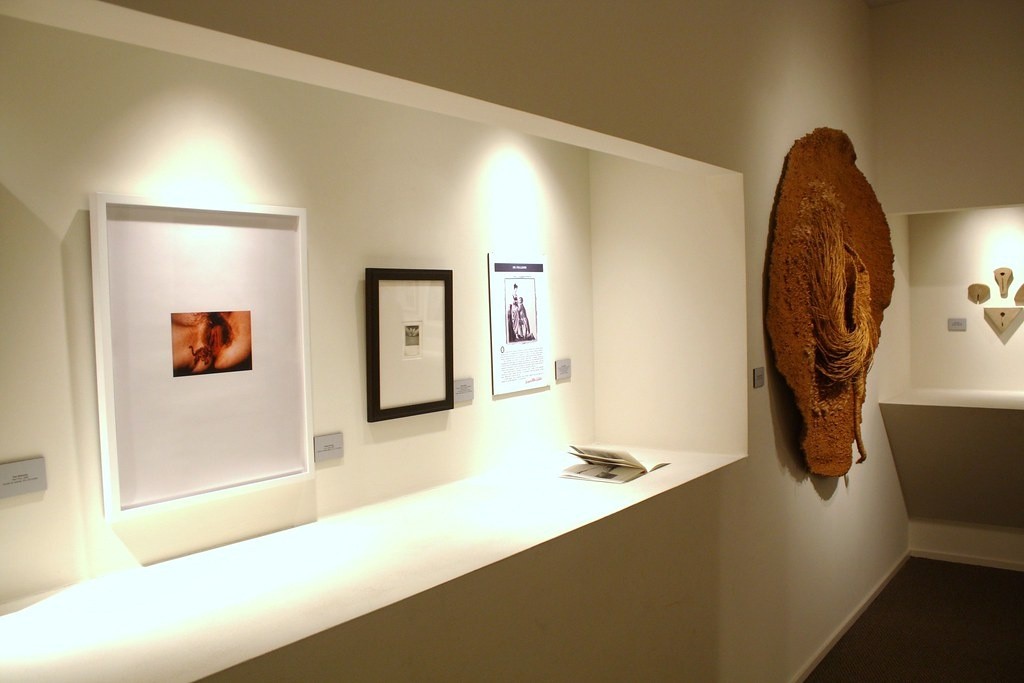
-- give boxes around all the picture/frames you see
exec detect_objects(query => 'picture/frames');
[365,268,454,423]
[87,191,315,524]
